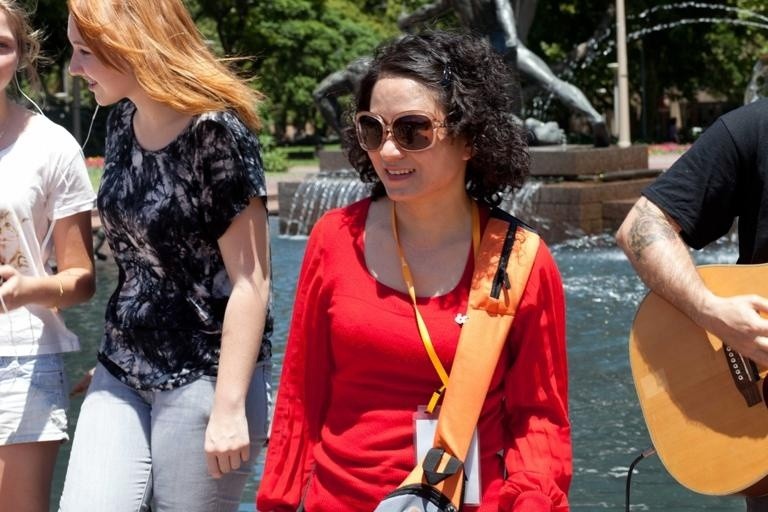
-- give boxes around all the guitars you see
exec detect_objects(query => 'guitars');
[629,262,767,497]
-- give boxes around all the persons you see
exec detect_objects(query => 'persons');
[311,53,371,144]
[615,93,768,510]
[1,1,98,512]
[251,27,572,510]
[472,0,610,147]
[56,0,276,511]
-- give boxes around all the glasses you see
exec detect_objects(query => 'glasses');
[350,112,454,154]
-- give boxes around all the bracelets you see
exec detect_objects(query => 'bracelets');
[46,274,64,310]
